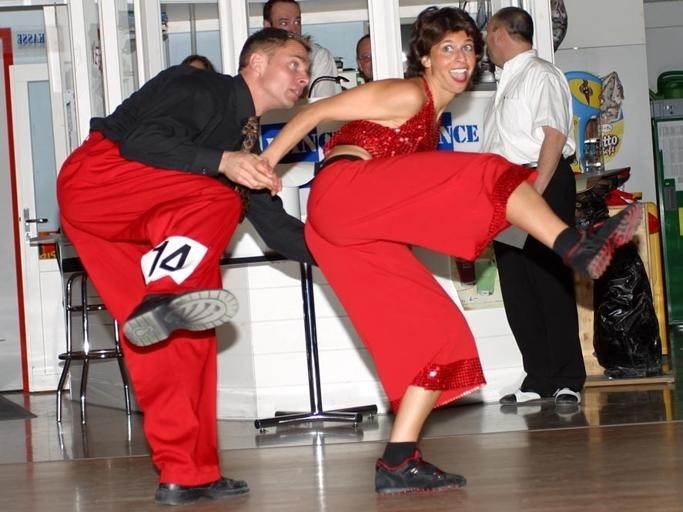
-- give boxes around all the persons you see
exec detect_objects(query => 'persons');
[180,54,215,72]
[262,0,343,105]
[355,34,373,83]
[258,6,643,496]
[478,6,588,407]
[56,28,318,505]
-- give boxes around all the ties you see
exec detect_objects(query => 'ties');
[232,117,259,223]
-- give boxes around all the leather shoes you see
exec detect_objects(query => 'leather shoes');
[123,289,239,348]
[154,477,251,506]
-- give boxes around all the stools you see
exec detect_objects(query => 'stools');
[56,257,131,424]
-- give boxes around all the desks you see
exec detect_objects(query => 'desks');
[220,253,378,433]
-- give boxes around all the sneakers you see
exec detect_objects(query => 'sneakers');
[499,389,551,408]
[374,448,467,499]
[553,387,581,406]
[562,201,644,281]
